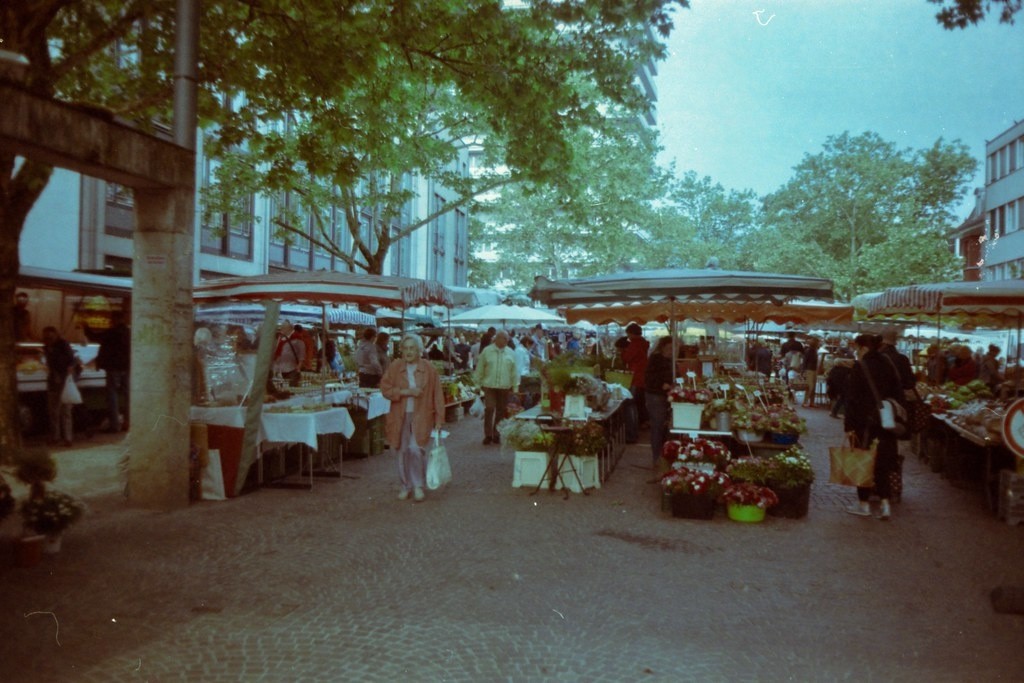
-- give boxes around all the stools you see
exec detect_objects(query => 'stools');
[530,414,589,498]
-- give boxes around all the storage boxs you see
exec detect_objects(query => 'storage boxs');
[999,469,1024,521]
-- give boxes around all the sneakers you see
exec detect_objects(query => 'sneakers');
[846,501,873,516]
[873,505,891,520]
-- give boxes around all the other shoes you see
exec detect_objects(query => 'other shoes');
[398,489,412,500]
[829,412,842,419]
[802,402,808,407]
[482,436,492,445]
[810,402,816,407]
[646,472,665,483]
[414,488,426,501]
[492,435,499,444]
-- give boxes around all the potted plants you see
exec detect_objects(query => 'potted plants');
[544,362,578,409]
[441,380,457,403]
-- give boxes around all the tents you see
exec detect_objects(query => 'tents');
[527,268,1024,383]
[193,268,452,470]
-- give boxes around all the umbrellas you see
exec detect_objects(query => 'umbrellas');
[441,303,565,330]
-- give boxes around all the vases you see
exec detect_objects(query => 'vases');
[769,483,811,516]
[672,403,705,430]
[717,412,730,431]
[756,430,800,444]
[727,498,766,522]
[679,492,718,517]
[511,424,625,493]
[737,428,764,441]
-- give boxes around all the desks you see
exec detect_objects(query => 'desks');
[670,428,735,437]
[930,412,1009,488]
[816,376,828,405]
[258,406,356,488]
[351,386,393,419]
[444,395,485,425]
[513,398,624,493]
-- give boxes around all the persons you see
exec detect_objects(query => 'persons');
[356,328,471,388]
[471,324,611,445]
[191,319,346,407]
[826,328,915,520]
[926,344,1008,396]
[79,311,131,434]
[13,293,34,357]
[617,323,650,445]
[646,336,681,470]
[42,326,78,444]
[750,332,819,408]
[380,334,445,501]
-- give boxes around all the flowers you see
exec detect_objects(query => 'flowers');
[661,374,816,509]
[498,409,622,456]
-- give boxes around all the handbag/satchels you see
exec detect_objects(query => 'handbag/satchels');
[908,385,931,432]
[61,374,82,404]
[877,398,907,435]
[829,432,876,488]
[426,429,452,490]
